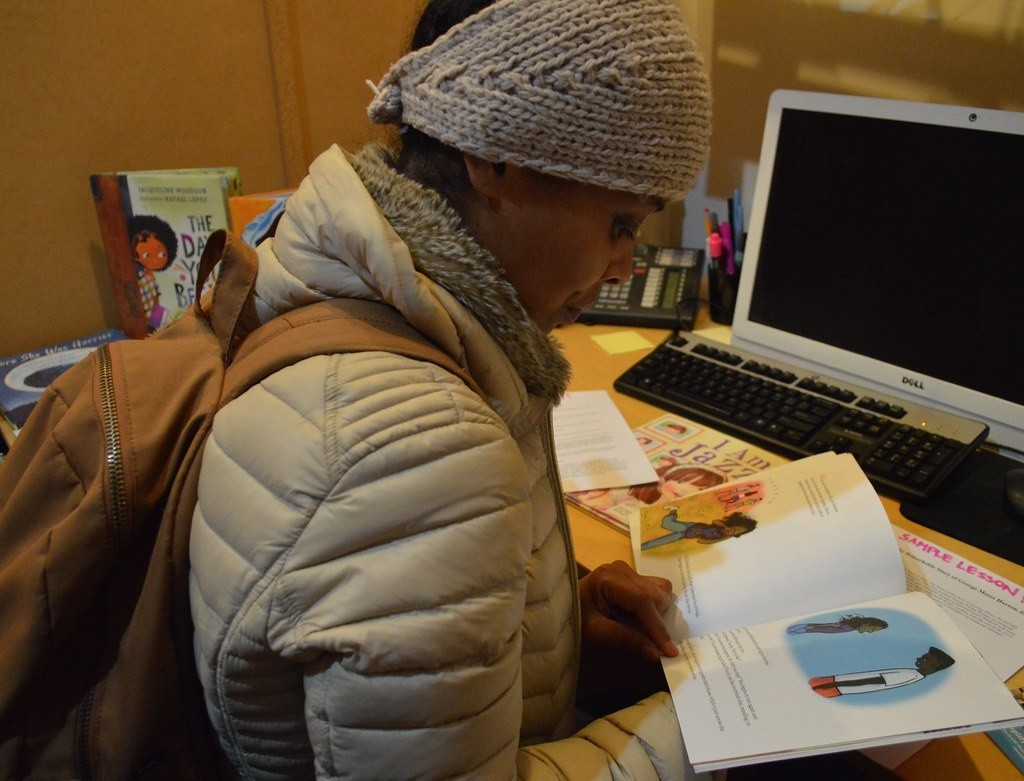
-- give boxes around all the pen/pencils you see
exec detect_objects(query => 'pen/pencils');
[705,188,747,270]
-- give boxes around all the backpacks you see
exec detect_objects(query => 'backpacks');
[0,228,498,781]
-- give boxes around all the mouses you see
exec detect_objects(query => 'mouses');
[1004,468,1024,518]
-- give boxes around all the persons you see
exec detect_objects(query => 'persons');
[190,0,730,781]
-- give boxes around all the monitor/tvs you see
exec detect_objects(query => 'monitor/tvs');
[728,90,1024,463]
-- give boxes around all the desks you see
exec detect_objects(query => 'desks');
[548,278,1024,781]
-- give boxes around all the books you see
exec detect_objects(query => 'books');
[0,167,300,438]
[561,412,1024,773]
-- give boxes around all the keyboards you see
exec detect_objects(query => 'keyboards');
[612,329,990,503]
